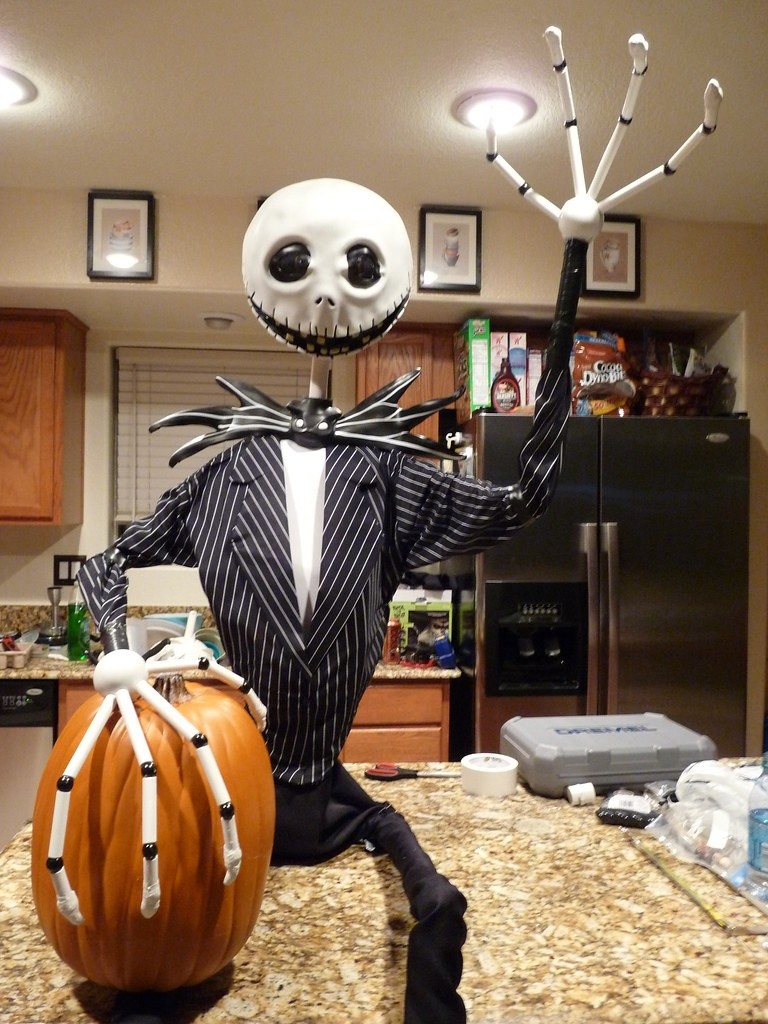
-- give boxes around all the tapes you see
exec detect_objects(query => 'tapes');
[461,753,519,797]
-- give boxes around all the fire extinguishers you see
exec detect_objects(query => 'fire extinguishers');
[383,618,401,664]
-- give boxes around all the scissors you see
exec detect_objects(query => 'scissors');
[365,762,462,781]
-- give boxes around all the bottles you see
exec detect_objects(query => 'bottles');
[68,573,90,662]
[749,751,768,901]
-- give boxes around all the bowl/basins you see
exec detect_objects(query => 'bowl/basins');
[146,612,202,631]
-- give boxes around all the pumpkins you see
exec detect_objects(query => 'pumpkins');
[29,673,276,993]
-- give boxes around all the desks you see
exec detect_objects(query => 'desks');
[0,755,768,1024]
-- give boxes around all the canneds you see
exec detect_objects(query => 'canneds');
[383,618,401,664]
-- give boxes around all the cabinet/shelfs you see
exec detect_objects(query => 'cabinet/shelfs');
[0,308,91,532]
[338,680,448,763]
[356,331,454,462]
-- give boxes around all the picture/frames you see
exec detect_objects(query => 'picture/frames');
[86,190,156,279]
[416,202,483,295]
[580,211,644,300]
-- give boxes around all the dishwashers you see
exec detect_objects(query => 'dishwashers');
[0,681,57,852]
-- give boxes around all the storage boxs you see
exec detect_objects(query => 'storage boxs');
[497,712,717,799]
[453,318,490,421]
[490,331,508,389]
[509,333,527,409]
[526,349,542,406]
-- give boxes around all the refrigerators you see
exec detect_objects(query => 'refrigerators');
[445,412,748,761]
